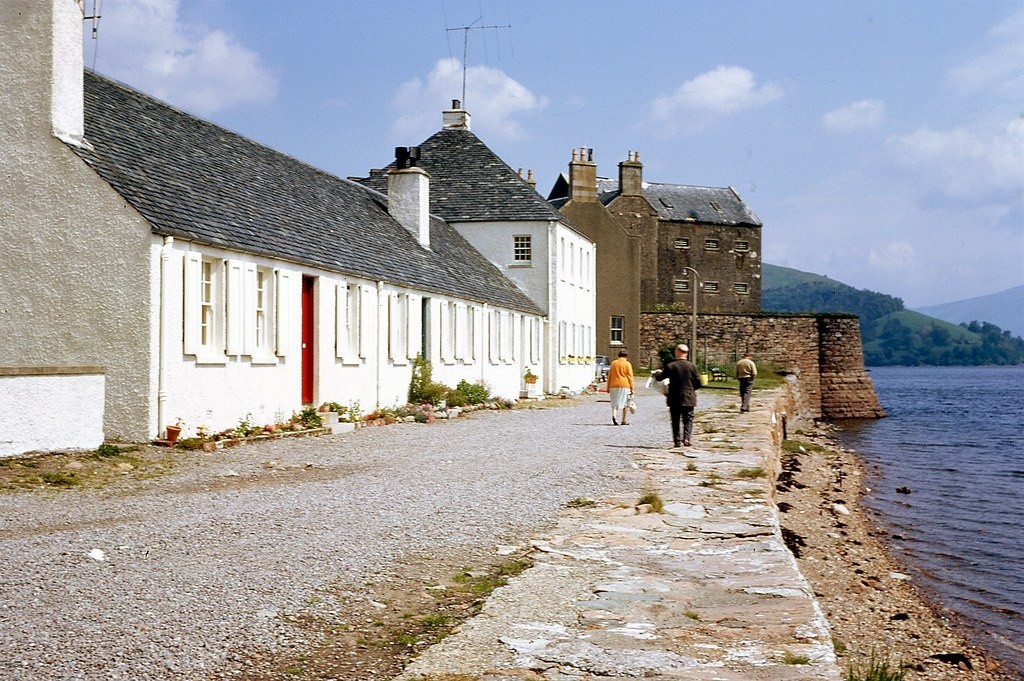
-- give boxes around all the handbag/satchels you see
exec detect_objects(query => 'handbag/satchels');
[628,398,636,414]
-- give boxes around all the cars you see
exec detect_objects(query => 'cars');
[596,355,611,382]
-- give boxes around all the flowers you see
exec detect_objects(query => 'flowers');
[523,366,539,379]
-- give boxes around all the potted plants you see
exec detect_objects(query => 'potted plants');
[166,418,185,443]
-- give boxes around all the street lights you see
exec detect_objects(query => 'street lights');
[681,265,701,366]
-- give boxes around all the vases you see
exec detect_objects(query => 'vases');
[526,378,536,383]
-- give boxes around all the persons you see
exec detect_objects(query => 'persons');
[655,344,702,448]
[607,348,634,426]
[733,352,758,413]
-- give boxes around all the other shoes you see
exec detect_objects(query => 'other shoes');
[674,442,680,447]
[621,422,630,425]
[682,438,691,446]
[613,416,618,425]
[740,408,749,413]
